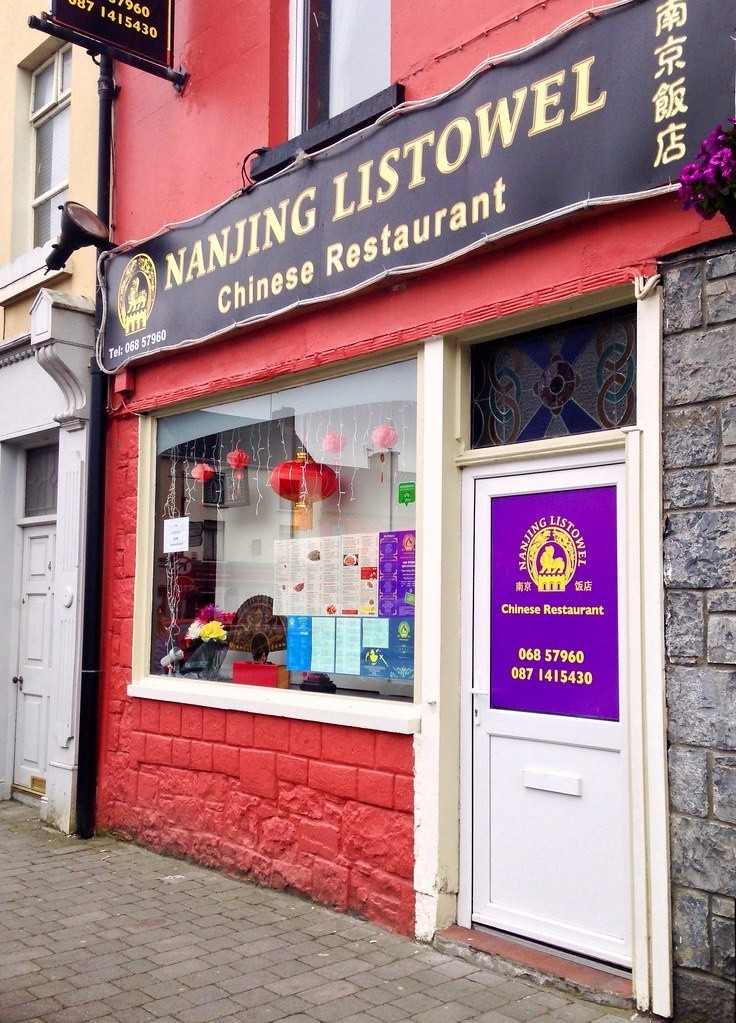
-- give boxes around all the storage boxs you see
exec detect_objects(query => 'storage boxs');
[233,662,289,688]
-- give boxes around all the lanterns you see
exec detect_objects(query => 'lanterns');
[372,427,399,484]
[226,450,251,480]
[324,429,346,467]
[191,463,214,483]
[270,453,338,531]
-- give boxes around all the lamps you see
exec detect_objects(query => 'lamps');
[161,647,183,676]
[43,199,118,271]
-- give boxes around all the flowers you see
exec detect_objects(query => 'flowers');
[675,117,736,232]
[184,604,228,644]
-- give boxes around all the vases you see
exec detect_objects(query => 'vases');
[195,646,228,679]
